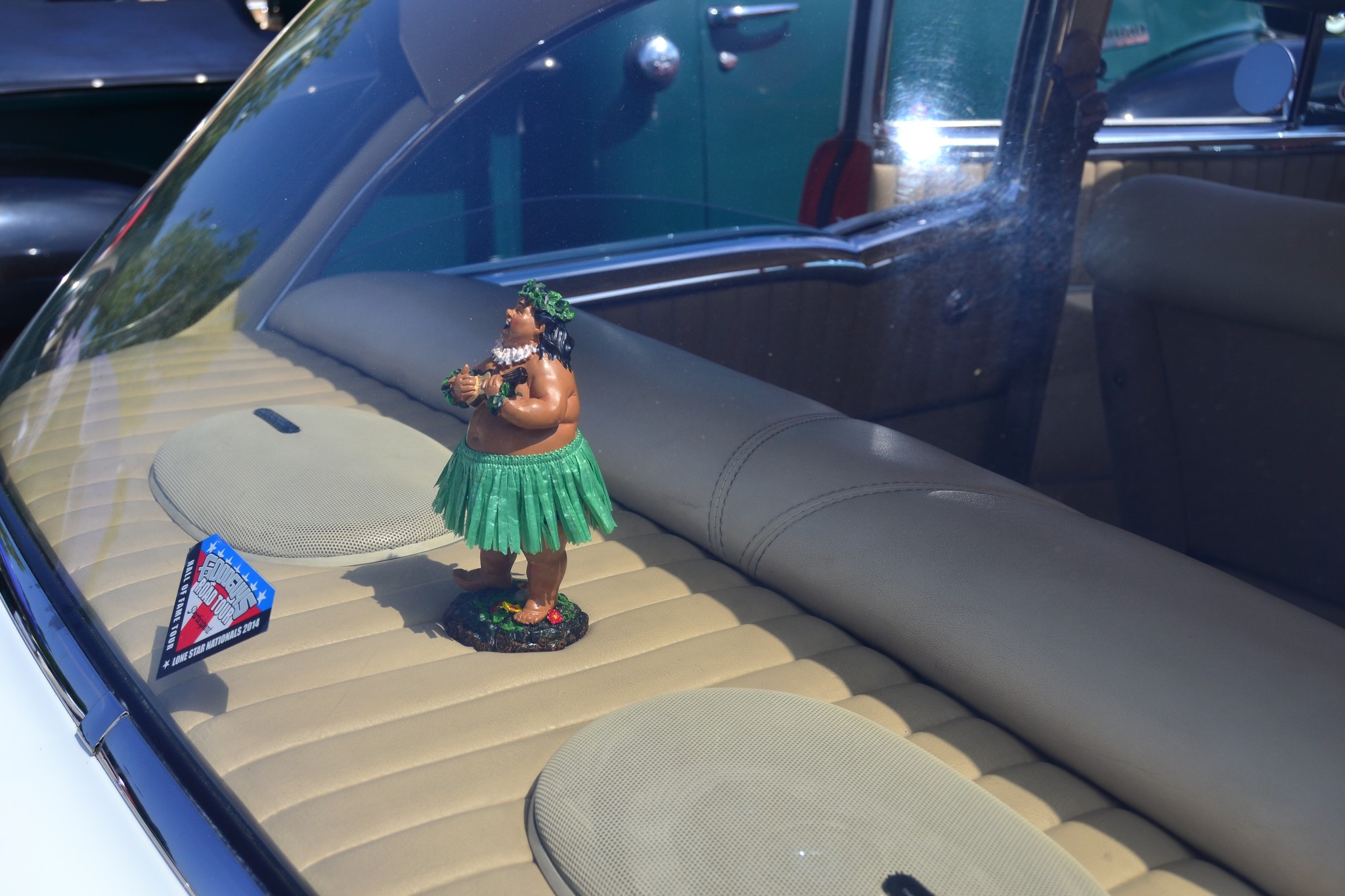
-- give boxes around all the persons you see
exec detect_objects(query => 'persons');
[428,281,615,625]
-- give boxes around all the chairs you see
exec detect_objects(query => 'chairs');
[1085,170,1345,616]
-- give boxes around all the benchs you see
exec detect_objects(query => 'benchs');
[272,269,1345,896]
